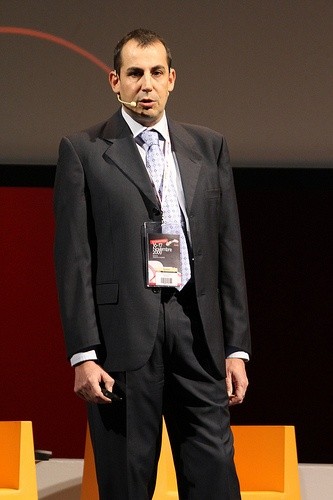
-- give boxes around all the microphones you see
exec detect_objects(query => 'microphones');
[117,94,136,108]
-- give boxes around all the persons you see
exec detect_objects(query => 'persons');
[52,28,252,500]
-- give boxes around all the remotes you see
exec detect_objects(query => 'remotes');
[99,381,118,401]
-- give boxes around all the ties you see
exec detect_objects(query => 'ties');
[138,129,193,291]
